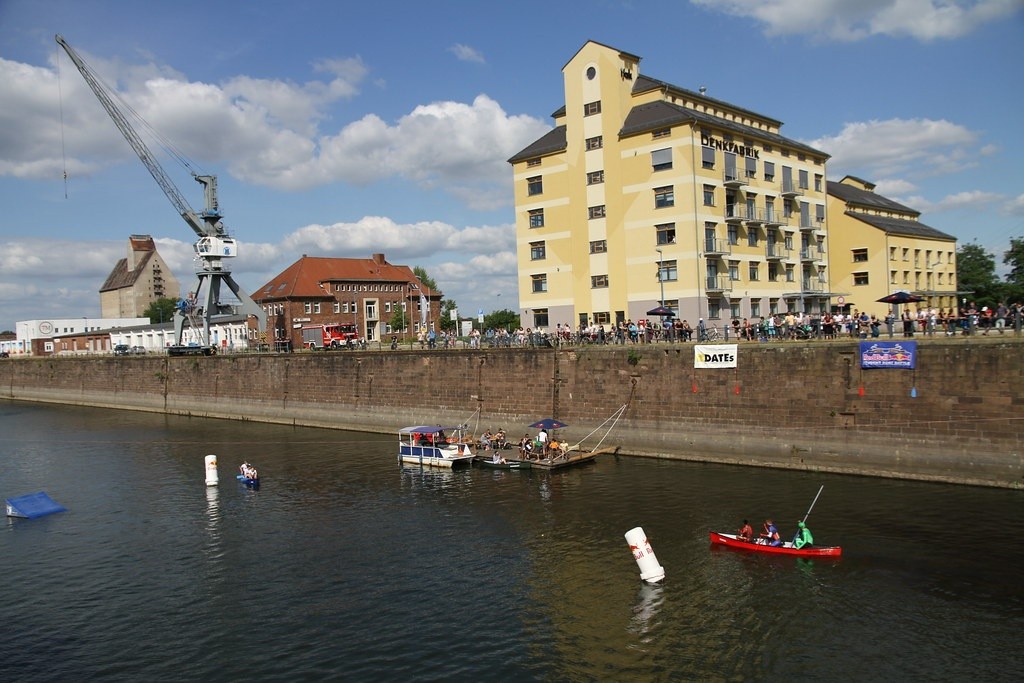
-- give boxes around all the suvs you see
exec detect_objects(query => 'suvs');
[129,346,146,355]
[0,353,9,359]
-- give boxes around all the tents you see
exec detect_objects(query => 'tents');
[875,292,927,319]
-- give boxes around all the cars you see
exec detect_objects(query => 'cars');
[114,345,131,355]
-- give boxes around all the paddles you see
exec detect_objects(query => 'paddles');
[790,485,824,547]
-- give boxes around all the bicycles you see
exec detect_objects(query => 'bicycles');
[698,322,724,343]
[794,328,823,340]
[567,333,615,347]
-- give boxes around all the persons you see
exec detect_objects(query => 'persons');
[699,318,704,343]
[240,460,257,479]
[230,341,234,354]
[464,424,469,437]
[580,316,693,345]
[346,338,352,350]
[414,432,427,446]
[480,428,507,451]
[213,344,218,352]
[417,328,456,349]
[732,309,882,342]
[467,323,570,348]
[884,306,957,338]
[518,429,569,460]
[738,520,814,550]
[959,302,1024,336]
[492,451,507,465]
[391,335,397,350]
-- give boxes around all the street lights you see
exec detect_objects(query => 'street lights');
[497,294,501,312]
[25,323,28,356]
[656,248,665,321]
[83,316,88,355]
[415,276,423,330]
[963,299,967,309]
[931,261,941,307]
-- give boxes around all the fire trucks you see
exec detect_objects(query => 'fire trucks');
[301,322,360,351]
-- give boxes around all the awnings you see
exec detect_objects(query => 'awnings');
[783,292,850,300]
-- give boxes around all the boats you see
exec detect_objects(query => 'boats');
[397,425,476,468]
[710,532,842,558]
[473,457,532,469]
[236,473,260,485]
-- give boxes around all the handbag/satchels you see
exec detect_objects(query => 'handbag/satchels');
[686,324,693,333]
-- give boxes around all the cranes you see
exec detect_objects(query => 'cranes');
[55,34,269,352]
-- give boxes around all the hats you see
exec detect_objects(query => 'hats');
[981,307,988,311]
[699,318,703,320]
[246,464,252,467]
[799,522,806,527]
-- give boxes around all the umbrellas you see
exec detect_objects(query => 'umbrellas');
[647,306,676,325]
[528,418,569,441]
